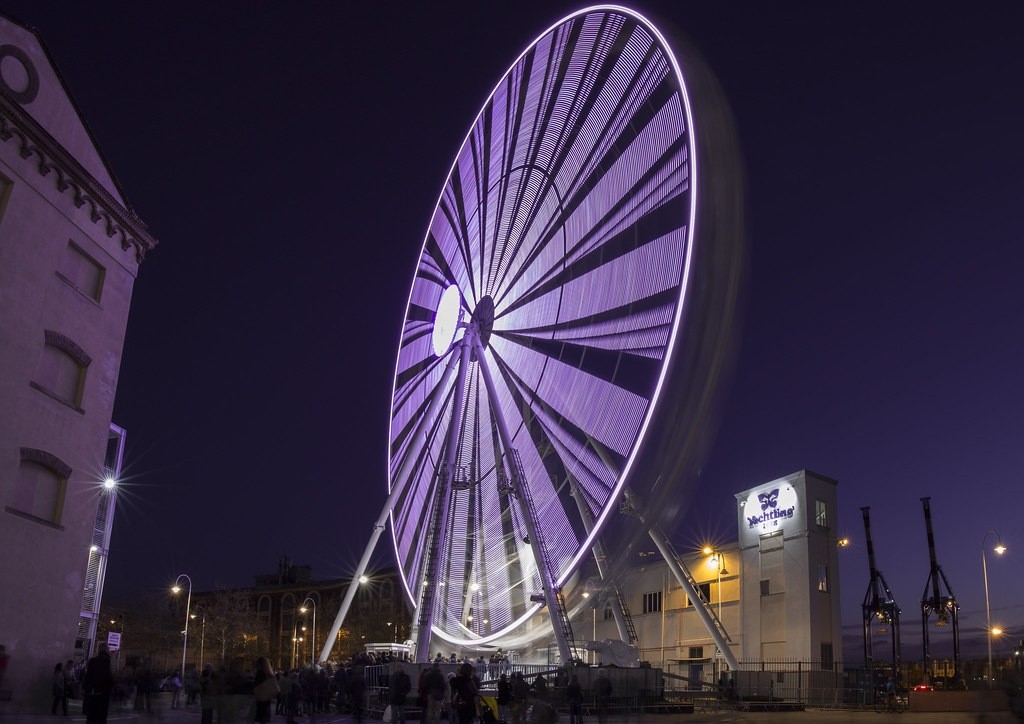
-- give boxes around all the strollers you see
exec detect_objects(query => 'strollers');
[474,695,508,724]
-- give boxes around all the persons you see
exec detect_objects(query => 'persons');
[886,676,895,711]
[47,638,614,724]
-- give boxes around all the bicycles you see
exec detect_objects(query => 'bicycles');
[874,688,907,713]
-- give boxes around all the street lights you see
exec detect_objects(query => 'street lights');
[190,605,205,676]
[705,553,723,682]
[171,574,192,688]
[292,619,306,669]
[300,598,316,667]
[982,528,1008,690]
[109,611,124,670]
